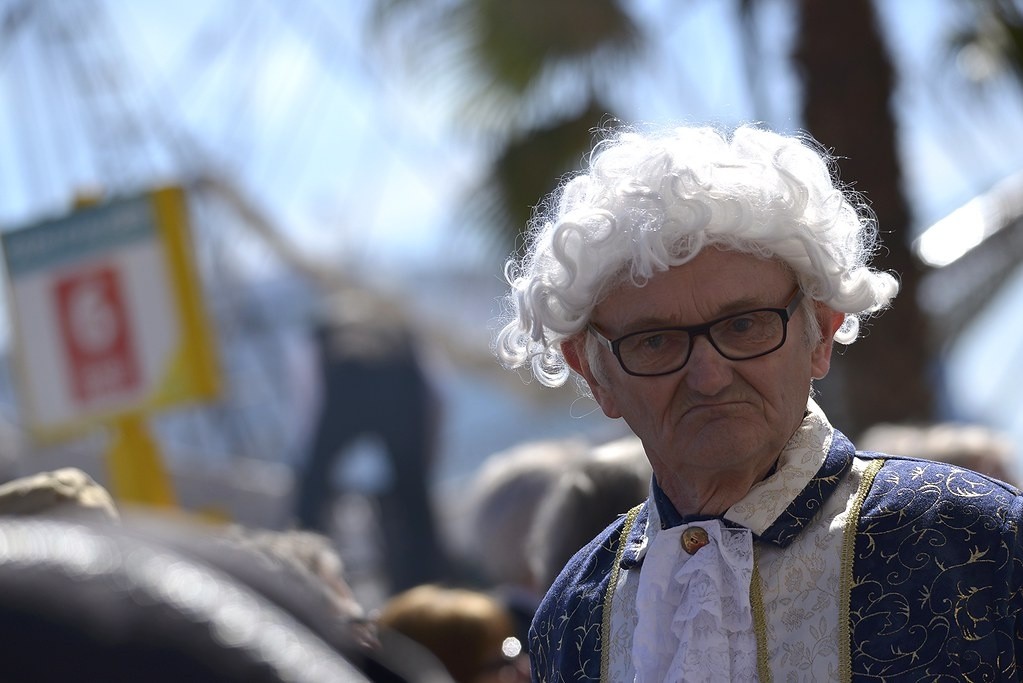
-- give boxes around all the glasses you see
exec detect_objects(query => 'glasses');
[586,286,804,377]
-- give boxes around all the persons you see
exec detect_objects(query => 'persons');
[0,119,1023,683]
